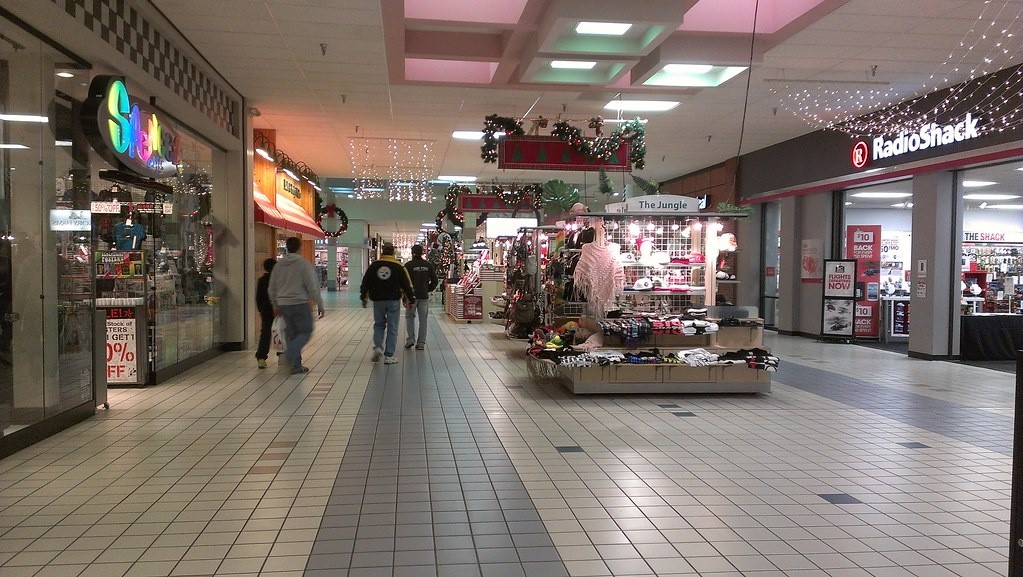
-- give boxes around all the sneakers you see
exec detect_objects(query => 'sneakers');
[372,346,383,362]
[384,355,398,363]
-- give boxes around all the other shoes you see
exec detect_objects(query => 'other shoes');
[290,365,309,373]
[416,344,424,350]
[405,338,414,347]
[258,358,267,369]
[278,355,286,364]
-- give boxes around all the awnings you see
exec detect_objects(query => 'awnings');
[253,193,325,239]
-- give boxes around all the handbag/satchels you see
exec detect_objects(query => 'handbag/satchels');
[514,302,534,323]
[271,316,287,353]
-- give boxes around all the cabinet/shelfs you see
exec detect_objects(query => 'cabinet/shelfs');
[520,212,776,395]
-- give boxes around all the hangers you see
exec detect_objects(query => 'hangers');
[113,213,143,229]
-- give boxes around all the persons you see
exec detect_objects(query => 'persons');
[361,243,416,366]
[269,238,325,373]
[402,246,438,350]
[255,258,287,369]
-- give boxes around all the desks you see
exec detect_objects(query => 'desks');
[881,295,985,344]
